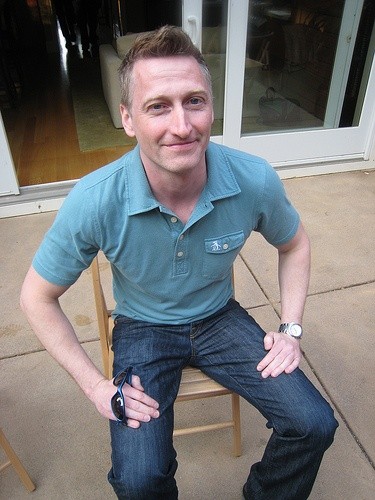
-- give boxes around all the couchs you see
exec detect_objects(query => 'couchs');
[100,27,264,128]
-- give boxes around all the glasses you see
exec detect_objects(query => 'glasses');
[111,366,133,426]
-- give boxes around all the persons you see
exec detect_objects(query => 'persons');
[77,0,100,59]
[53,0,79,43]
[19,24,339,500]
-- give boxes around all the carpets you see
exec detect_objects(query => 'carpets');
[72,84,134,152]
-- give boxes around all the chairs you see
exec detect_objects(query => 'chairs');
[92,255,243,456]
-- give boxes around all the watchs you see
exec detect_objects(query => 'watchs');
[278,323,303,340]
